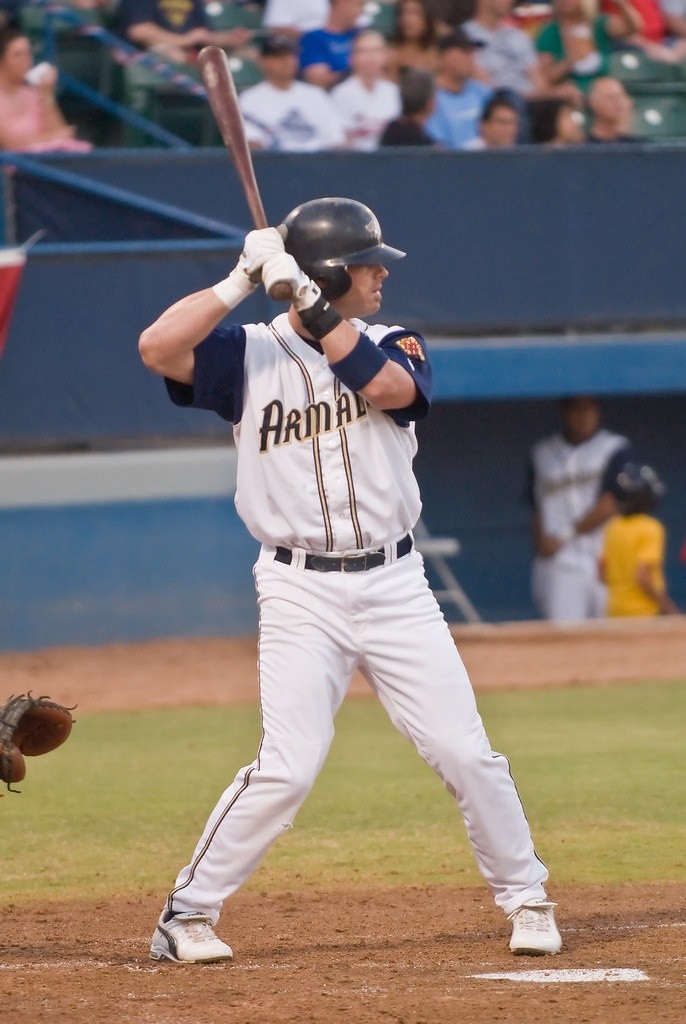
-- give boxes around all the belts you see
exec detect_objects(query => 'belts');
[274,533,413,574]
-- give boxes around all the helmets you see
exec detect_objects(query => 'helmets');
[280,197,406,299]
[610,464,662,508]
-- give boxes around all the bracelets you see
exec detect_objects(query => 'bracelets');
[328,332,388,393]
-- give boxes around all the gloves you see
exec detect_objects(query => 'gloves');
[261,253,321,312]
[229,224,288,295]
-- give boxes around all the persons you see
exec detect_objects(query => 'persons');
[598,465,681,618]
[329,31,402,151]
[534,0,686,92]
[424,32,491,149]
[529,99,582,146]
[523,397,635,621]
[392,0,440,74]
[0,0,263,173]
[136,197,561,963]
[298,0,364,90]
[237,36,344,151]
[585,77,656,144]
[461,0,540,97]
[262,1,330,32]
[479,103,519,149]
[381,74,443,147]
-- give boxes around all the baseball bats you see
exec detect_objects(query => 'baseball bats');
[198,45,293,302]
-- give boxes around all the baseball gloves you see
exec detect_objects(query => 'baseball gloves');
[0,695,73,783]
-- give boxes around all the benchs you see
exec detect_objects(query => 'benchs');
[18,0,686,150]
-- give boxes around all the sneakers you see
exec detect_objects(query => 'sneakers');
[149,908,232,963]
[506,902,562,956]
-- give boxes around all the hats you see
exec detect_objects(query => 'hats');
[260,32,304,54]
[438,31,487,48]
